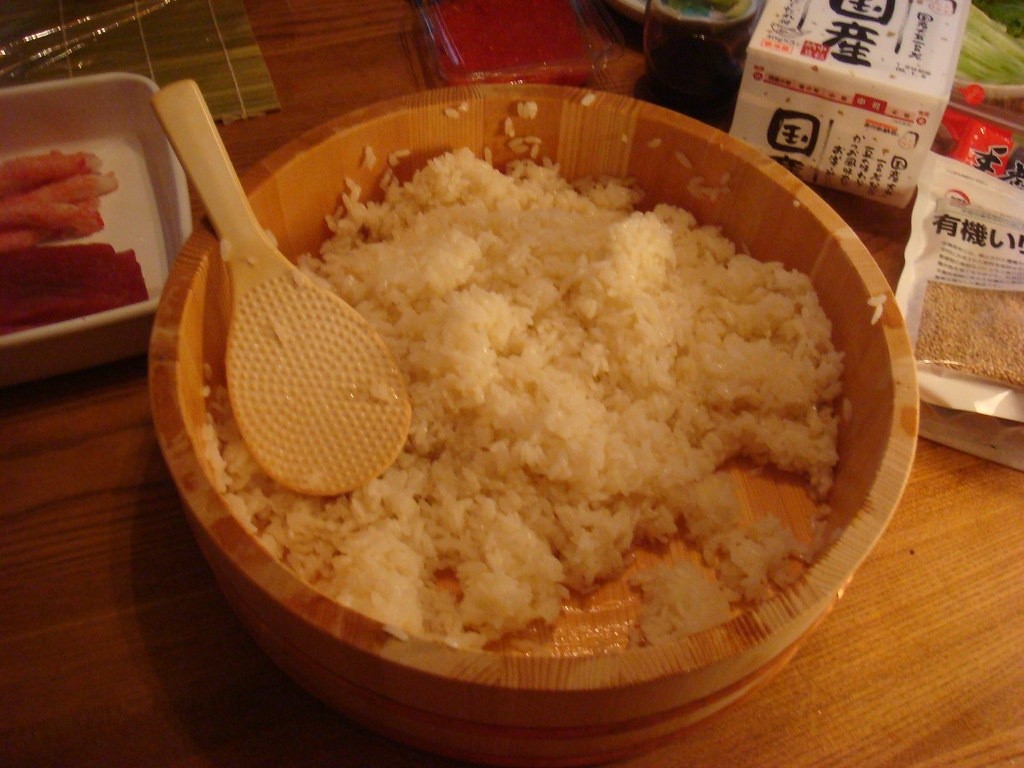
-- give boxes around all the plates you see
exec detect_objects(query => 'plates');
[0,72,191,382]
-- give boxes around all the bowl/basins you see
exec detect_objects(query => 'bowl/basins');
[145,81,918,767]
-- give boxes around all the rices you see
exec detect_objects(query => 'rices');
[188,96,887,648]
